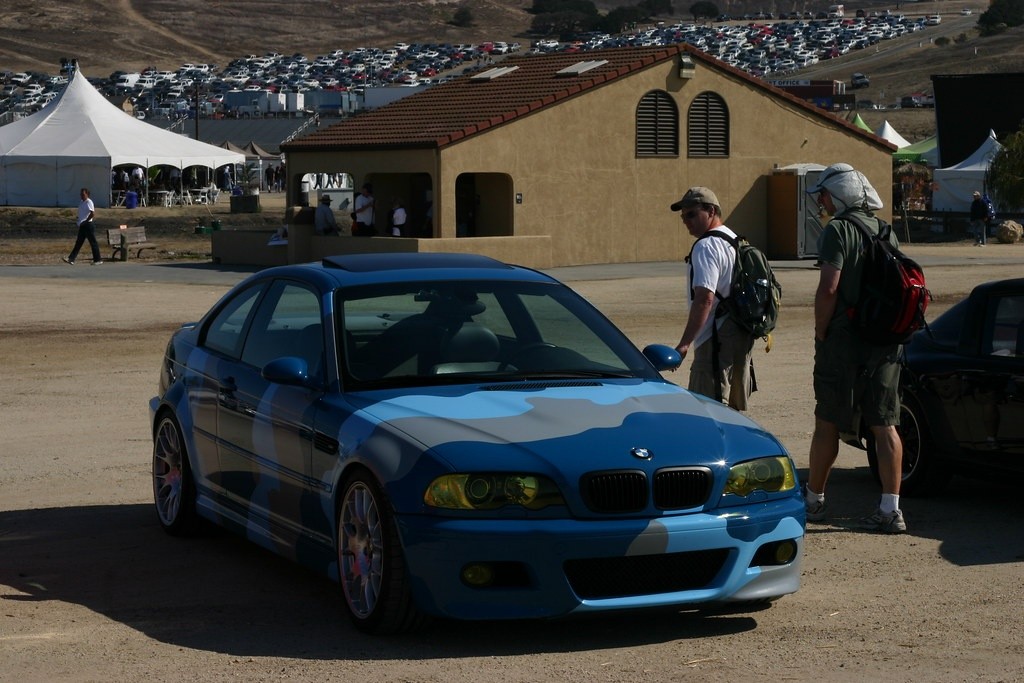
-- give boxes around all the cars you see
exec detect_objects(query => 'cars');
[0,40,521,124]
[866,278,1024,499]
[149,252,806,637]
[524,4,973,75]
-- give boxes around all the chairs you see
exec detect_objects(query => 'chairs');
[109,187,224,207]
[295,323,358,376]
[431,323,519,375]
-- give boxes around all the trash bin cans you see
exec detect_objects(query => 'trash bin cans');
[126,191,137,209]
[229,195,260,211]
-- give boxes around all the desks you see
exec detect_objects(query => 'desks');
[190,188,208,205]
[148,190,169,206]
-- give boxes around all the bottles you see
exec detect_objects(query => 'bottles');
[740,269,760,306]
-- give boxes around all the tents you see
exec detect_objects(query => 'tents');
[0,62,246,208]
[931,136,1024,233]
[851,113,938,168]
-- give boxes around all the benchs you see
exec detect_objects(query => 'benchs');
[107,226,160,258]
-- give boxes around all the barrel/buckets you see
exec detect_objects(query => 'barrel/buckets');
[212,220,222,231]
[125,192,138,209]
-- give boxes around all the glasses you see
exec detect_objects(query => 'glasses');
[681,206,706,221]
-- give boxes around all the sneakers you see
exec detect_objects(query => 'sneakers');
[857,508,906,532]
[801,481,824,520]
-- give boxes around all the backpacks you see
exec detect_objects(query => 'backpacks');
[815,213,927,346]
[685,225,781,339]
[982,194,996,220]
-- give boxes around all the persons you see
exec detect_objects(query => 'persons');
[812,194,835,267]
[422,191,476,235]
[112,164,233,195]
[798,162,906,535]
[264,161,286,193]
[62,188,104,265]
[668,187,759,410]
[350,183,378,236]
[311,172,354,189]
[386,195,411,237]
[314,195,341,236]
[970,190,988,247]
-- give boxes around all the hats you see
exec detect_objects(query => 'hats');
[972,191,980,196]
[319,195,333,202]
[671,186,722,218]
[806,186,824,193]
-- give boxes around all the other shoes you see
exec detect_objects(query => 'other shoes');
[91,260,104,265]
[62,258,73,264]
